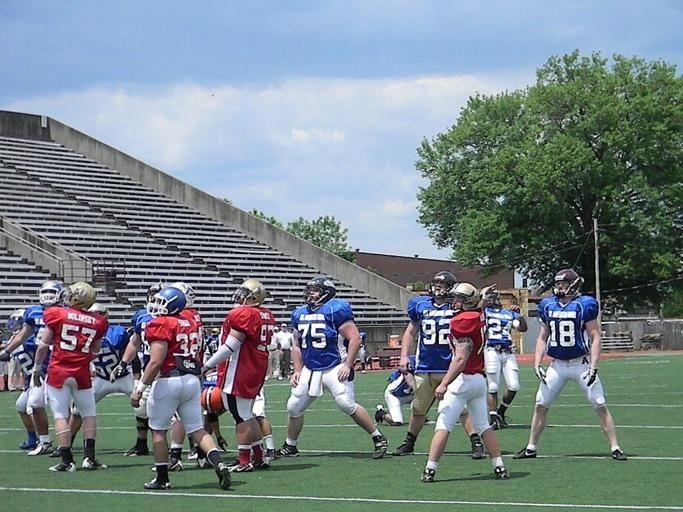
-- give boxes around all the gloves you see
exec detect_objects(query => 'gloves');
[534,365,546,385]
[110,361,126,383]
[32,366,45,386]
[483,284,497,300]
[583,367,597,386]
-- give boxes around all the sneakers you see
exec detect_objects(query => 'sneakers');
[20,439,107,471]
[152,445,299,472]
[473,442,536,479]
[144,478,170,490]
[216,462,231,489]
[375,404,383,423]
[421,468,435,482]
[490,415,508,430]
[123,448,149,456]
[373,435,413,459]
[611,447,626,460]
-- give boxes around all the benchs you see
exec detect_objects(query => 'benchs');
[370,348,401,370]
[600,337,633,352]
[0,136,425,326]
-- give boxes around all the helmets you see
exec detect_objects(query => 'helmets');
[231,280,265,307]
[146,282,195,315]
[553,269,584,298]
[38,280,95,309]
[429,271,480,311]
[303,278,335,306]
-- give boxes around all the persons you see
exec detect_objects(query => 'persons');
[268,323,294,381]
[391,271,487,459]
[479,284,528,430]
[420,283,510,482]
[0,282,65,456]
[375,356,415,426]
[275,276,390,460]
[513,268,627,461]
[50,303,145,457]
[32,282,108,473]
[200,279,276,472]
[109,281,232,491]
